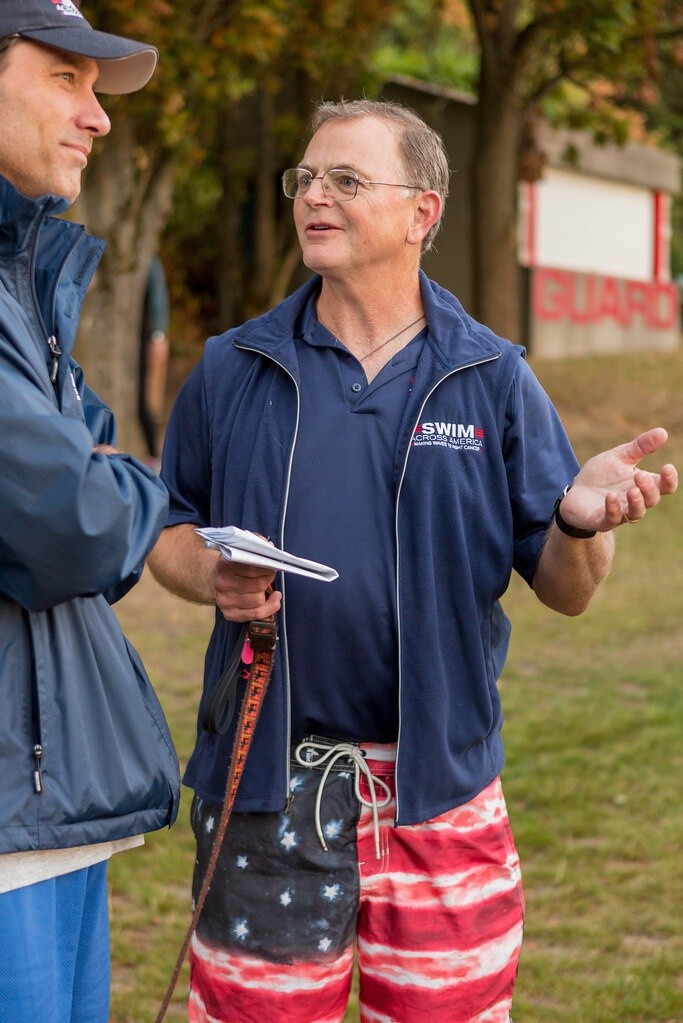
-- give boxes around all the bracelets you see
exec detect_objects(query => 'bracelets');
[550,482,599,539]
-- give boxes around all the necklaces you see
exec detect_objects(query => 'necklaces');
[334,306,433,369]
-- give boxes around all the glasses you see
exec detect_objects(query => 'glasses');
[281,168,428,201]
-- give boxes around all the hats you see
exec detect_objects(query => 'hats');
[1,1,160,95]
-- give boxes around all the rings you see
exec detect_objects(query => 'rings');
[623,502,641,523]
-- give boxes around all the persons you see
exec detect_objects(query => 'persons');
[0,2,182,1023]
[151,100,671,1023]
